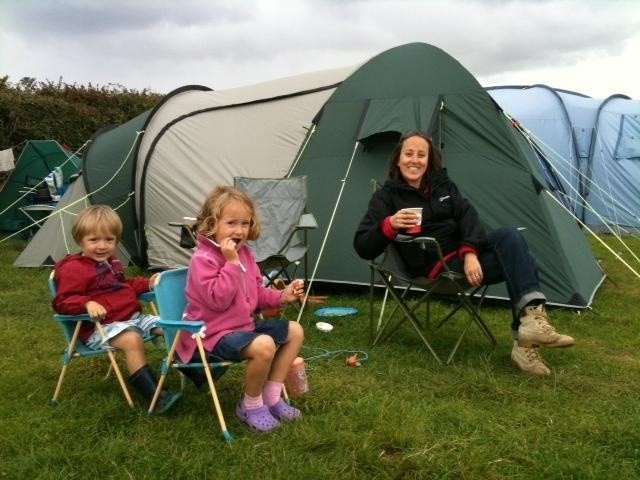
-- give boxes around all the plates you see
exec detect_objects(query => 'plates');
[312,305,359,318]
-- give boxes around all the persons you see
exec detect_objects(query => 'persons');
[53,203,232,413]
[174,184,306,434]
[350,129,576,378]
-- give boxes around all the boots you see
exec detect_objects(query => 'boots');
[517,307,574,348]
[178,364,227,391]
[512,339,550,375]
[127,364,183,417]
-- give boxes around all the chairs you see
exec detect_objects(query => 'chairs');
[148,266,290,442]
[168,176,318,321]
[369,179,503,365]
[50,270,184,413]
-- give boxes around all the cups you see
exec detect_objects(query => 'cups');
[181,215,198,229]
[402,207,423,234]
[286,356,309,397]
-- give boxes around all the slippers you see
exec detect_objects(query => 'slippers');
[269,398,301,424]
[236,399,281,433]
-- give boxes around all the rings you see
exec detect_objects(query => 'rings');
[471,274,479,279]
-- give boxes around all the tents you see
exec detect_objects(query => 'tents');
[1,138,83,235]
[482,83,640,236]
[11,42,611,311]
